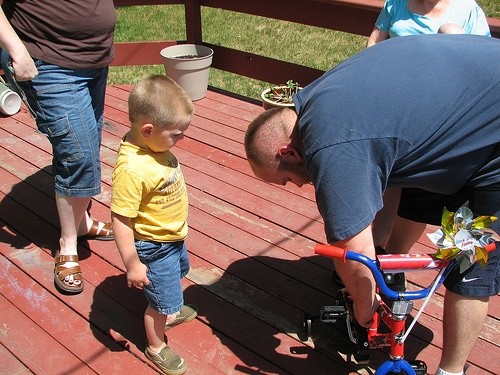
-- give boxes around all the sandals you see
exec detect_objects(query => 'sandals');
[55,247,83,292]
[77,220,116,240]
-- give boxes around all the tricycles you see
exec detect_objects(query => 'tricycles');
[302,243,460,375]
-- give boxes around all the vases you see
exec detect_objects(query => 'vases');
[160,44,214,102]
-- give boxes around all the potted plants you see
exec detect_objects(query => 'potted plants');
[260,80,304,110]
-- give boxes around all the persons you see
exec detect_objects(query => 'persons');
[1,0,116,292]
[244,34,500,375]
[111,74,197,375]
[368,0,491,52]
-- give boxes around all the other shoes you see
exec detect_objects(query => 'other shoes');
[333,262,406,291]
[164,305,197,331]
[145,345,186,375]
